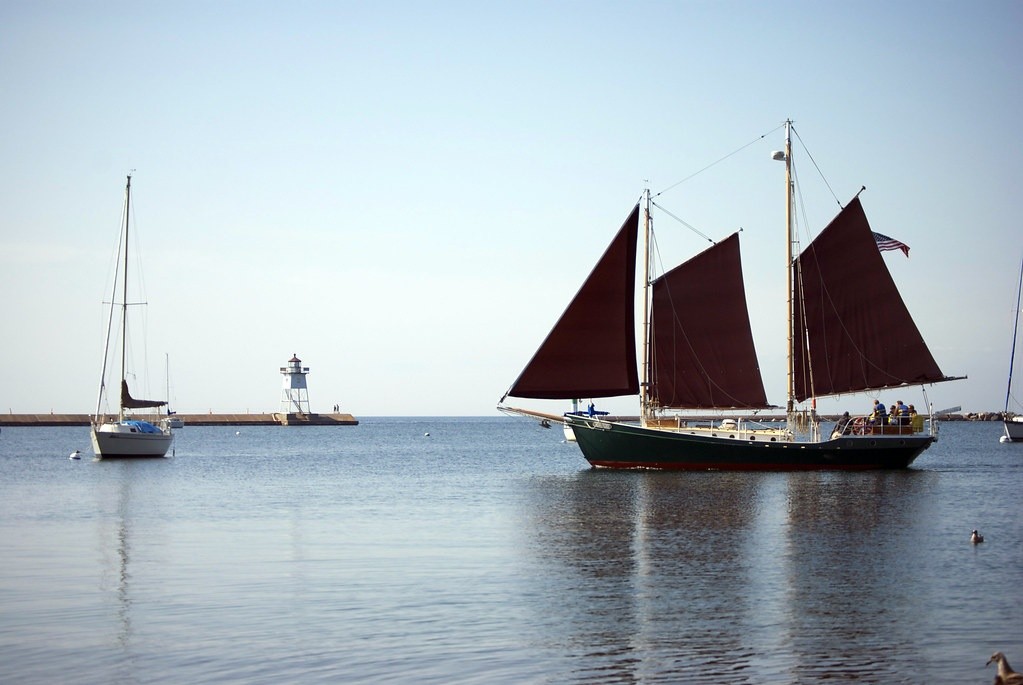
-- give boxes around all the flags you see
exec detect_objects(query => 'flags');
[871,232,910,257]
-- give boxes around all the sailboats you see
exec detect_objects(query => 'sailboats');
[999,258,1023,447]
[90,170,176,458]
[495,117,971,469]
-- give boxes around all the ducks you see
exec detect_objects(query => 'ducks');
[70,450,81,459]
[986,651,1023,685]
[970,530,984,542]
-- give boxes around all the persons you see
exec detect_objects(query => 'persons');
[334,404,339,414]
[831,412,857,439]
[890,401,917,425]
[873,399,887,425]
